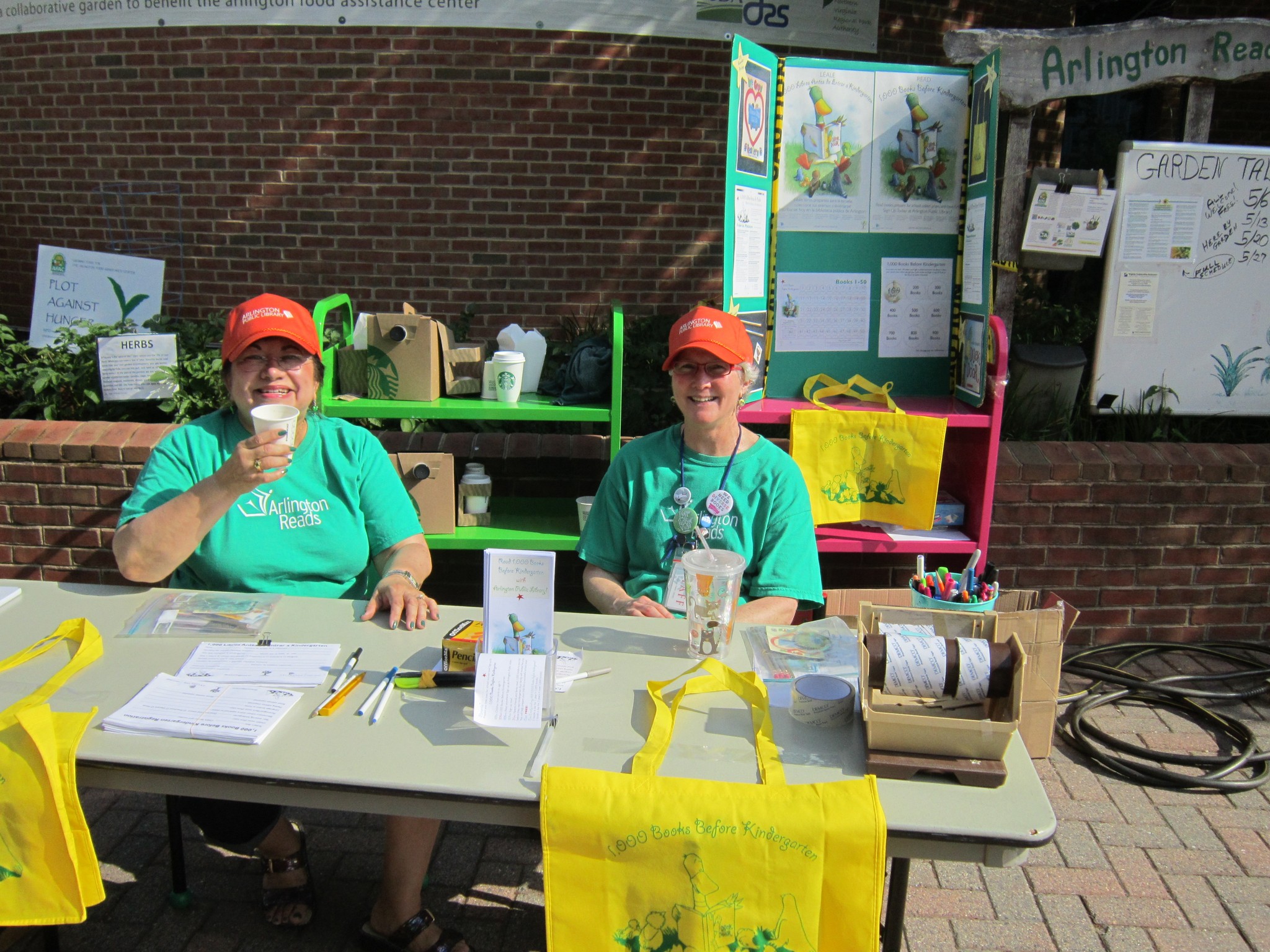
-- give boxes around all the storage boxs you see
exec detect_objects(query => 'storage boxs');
[337,341,368,398]
[386,448,455,536]
[441,619,485,672]
[818,579,1080,760]
[366,299,443,399]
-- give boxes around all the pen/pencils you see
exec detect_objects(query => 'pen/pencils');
[395,672,476,688]
[357,666,398,716]
[372,674,396,724]
[555,666,611,684]
[530,712,558,777]
[318,671,367,717]
[332,647,363,694]
[310,673,359,719]
[911,548,1000,603]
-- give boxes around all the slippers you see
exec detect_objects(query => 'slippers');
[361,907,476,952]
[261,817,316,929]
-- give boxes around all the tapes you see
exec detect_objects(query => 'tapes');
[877,621,946,699]
[952,636,992,704]
[788,673,856,728]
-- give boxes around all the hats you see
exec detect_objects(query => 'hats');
[222,293,322,368]
[662,306,754,370]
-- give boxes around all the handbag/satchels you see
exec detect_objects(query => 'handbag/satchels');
[787,372,948,531]
[0,617,109,928]
[537,656,886,951]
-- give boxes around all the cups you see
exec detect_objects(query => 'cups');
[460,474,492,514]
[682,549,747,660]
[464,462,486,474]
[492,351,526,402]
[480,361,497,399]
[250,404,300,468]
[575,496,598,535]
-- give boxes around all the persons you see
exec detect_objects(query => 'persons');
[575,306,824,626]
[112,291,474,952]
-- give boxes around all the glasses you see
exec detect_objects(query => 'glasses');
[672,360,746,377]
[235,352,317,371]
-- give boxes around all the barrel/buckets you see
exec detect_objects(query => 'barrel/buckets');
[909,572,999,612]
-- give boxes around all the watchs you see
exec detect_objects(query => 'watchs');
[382,569,419,591]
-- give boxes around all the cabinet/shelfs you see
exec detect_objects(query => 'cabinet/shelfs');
[736,314,1009,580]
[306,291,627,552]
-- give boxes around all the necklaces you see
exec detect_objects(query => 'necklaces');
[295,420,304,438]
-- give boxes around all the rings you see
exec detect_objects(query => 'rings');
[254,460,263,472]
[416,594,424,601]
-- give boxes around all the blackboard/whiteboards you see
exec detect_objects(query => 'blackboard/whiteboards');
[1088,139,1269,416]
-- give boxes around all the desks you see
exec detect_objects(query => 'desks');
[1,574,1060,952]
